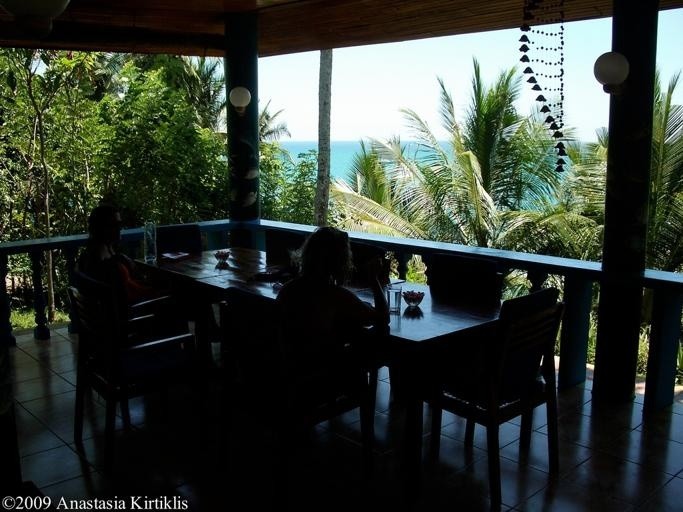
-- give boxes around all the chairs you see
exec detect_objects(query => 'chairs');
[423,286,567,512]
[348,240,390,287]
[219,287,379,436]
[67,273,195,439]
[428,252,498,314]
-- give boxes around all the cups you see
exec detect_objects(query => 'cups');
[386,286,402,316]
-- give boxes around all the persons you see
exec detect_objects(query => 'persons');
[79,203,219,334]
[276,226,390,326]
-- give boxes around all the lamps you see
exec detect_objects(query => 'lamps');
[594,50,630,96]
[228,86,253,114]
